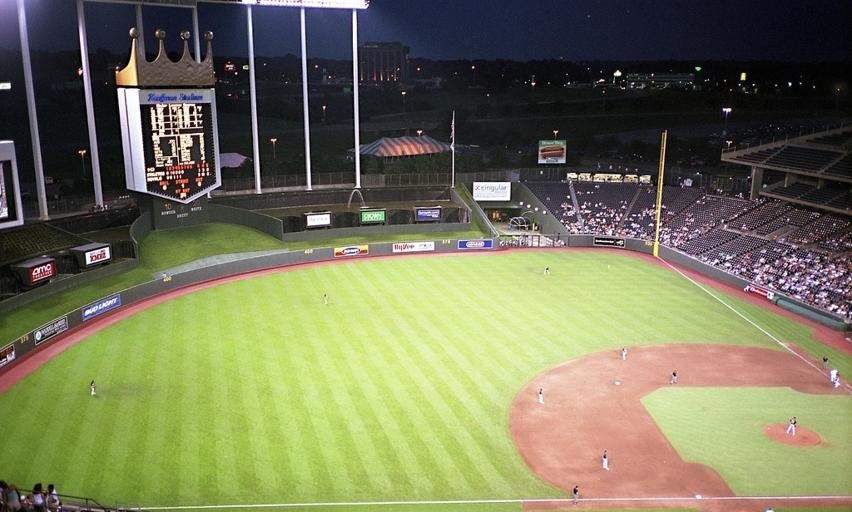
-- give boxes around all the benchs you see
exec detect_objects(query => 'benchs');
[525,179,714,266]
[714,129,852,322]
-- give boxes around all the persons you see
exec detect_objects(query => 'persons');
[669,367,678,383]
[822,355,829,368]
[0,479,64,512]
[830,367,840,389]
[572,485,578,504]
[538,388,544,404]
[602,449,609,470]
[90,379,96,396]
[622,344,628,361]
[324,293,328,304]
[544,266,549,275]
[786,416,798,436]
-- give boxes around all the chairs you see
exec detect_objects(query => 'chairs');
[0,224,90,264]
